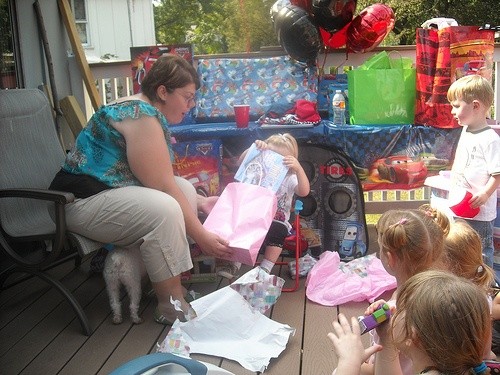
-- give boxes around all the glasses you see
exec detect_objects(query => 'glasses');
[168,88,195,102]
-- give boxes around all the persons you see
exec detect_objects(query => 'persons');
[327,202,500,375]
[235,132,310,274]
[448,75,500,267]
[46,51,234,324]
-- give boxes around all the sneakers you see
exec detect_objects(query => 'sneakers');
[217,264,240,279]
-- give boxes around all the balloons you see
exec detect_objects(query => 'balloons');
[269,0,396,65]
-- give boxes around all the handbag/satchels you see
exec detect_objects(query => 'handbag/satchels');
[348,50,417,124]
[416,17,494,128]
[201,162,277,266]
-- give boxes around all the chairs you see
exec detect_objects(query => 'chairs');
[229,196,300,294]
[0,88,102,337]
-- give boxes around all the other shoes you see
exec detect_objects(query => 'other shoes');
[153,290,203,303]
[154,307,197,325]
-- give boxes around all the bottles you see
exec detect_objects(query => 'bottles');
[332,90,345,126]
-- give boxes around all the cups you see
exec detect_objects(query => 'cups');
[234,105,250,128]
